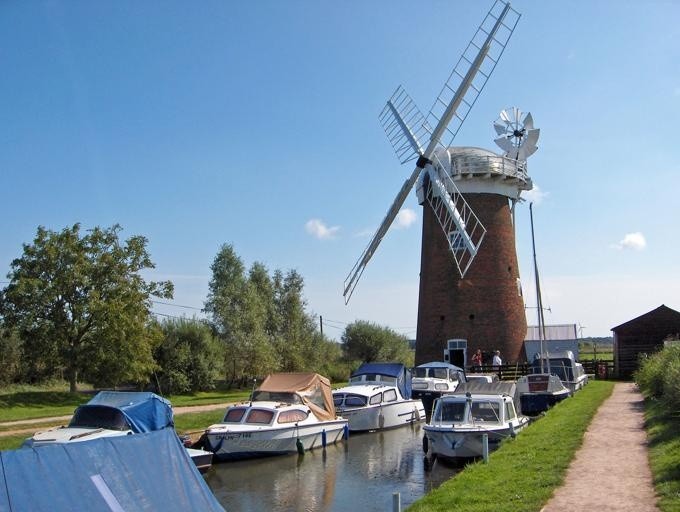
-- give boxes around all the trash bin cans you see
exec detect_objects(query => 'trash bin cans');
[598,364,607,378]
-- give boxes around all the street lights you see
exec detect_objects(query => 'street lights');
[20,426,213,474]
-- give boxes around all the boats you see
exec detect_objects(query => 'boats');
[330,385,426,431]
[411,362,501,395]
[422,351,588,472]
[204,401,349,459]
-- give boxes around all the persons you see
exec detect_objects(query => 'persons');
[472,350,482,371]
[492,351,502,372]
